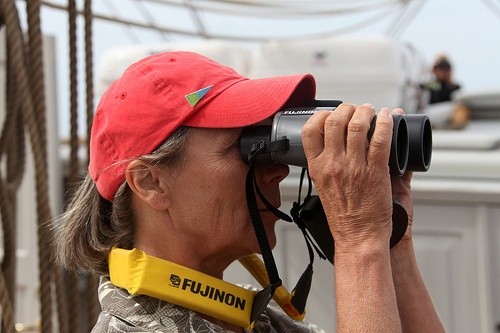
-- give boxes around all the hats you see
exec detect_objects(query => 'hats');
[87,50,319,203]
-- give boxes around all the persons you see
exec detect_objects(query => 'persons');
[420,55,460,105]
[56,50,446,332]
